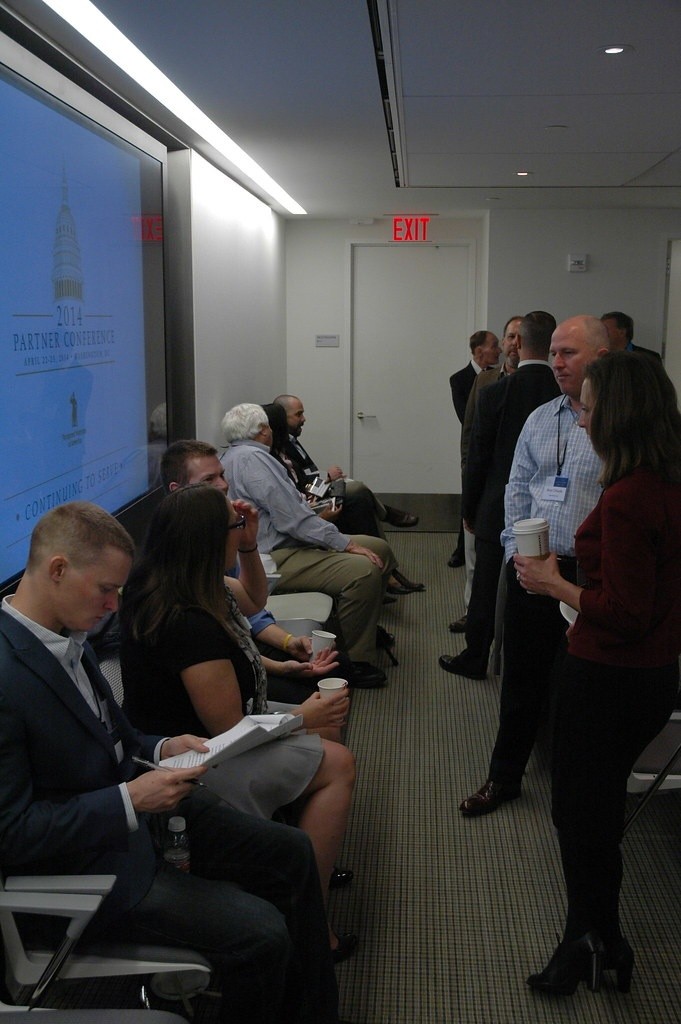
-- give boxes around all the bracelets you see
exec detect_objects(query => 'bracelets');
[347,542,355,553]
[238,544,257,554]
[283,634,293,653]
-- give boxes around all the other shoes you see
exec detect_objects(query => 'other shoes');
[322,661,388,689]
[385,585,414,594]
[438,654,487,681]
[448,615,468,633]
[375,625,395,647]
[382,597,398,604]
[447,548,465,567]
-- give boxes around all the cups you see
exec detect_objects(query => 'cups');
[511,518,551,593]
[318,678,348,723]
[309,629,335,664]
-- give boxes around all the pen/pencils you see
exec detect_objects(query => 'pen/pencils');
[132,755,206,789]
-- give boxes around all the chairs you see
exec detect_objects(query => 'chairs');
[0,872,210,1024]
[621,710,681,835]
[266,592,334,623]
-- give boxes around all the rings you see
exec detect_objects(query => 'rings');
[516,574,520,581]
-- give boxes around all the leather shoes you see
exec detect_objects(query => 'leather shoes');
[332,933,358,964]
[327,866,354,890]
[459,780,522,814]
[392,568,425,592]
[379,503,419,527]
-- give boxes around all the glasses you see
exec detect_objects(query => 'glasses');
[228,514,247,529]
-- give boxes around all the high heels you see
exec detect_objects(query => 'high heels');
[523,931,605,996]
[604,938,635,992]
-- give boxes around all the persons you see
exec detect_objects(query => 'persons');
[117,478,358,965]
[513,340,681,1000]
[259,394,425,604]
[0,497,342,1024]
[161,439,340,706]
[436,310,664,820]
[212,401,400,688]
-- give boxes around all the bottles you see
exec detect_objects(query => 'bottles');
[161,816,190,880]
